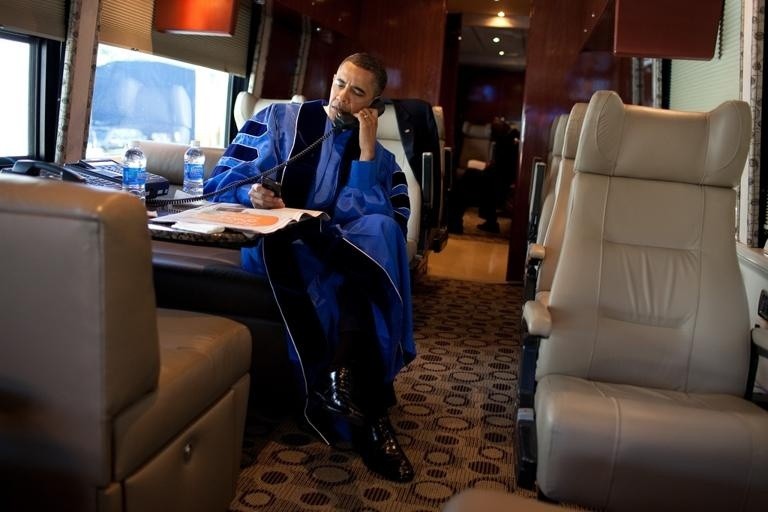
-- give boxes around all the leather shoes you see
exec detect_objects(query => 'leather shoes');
[314,366,363,420]
[361,420,414,483]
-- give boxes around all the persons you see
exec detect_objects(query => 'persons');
[200,50,422,486]
[442,110,521,236]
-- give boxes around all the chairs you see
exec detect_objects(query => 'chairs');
[456,119,521,179]
[515,90,768,512]
[1,170,250,512]
[132,91,449,458]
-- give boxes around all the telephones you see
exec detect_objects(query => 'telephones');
[336,97,385,132]
[2,159,122,190]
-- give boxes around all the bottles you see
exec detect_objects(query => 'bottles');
[183,140,205,198]
[122,140,146,208]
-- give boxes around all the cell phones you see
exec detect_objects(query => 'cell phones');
[260,175,283,200]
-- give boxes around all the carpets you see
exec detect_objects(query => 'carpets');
[449,209,511,244]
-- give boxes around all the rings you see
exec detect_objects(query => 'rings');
[363,114,369,119]
